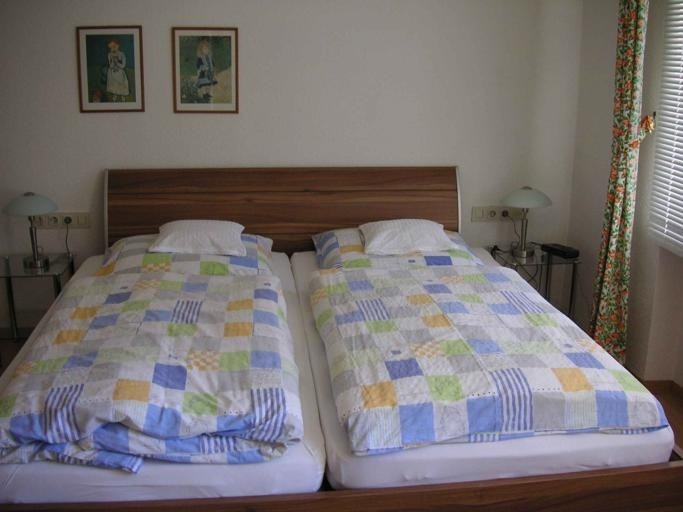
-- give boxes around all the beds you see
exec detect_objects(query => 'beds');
[0,167,683,512]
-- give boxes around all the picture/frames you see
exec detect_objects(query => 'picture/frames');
[74,25,238,115]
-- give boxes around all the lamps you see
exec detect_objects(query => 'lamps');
[501,186,553,258]
[2,192,57,268]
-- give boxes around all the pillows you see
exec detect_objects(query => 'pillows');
[100,217,274,278]
[309,219,482,277]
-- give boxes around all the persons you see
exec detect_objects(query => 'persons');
[104,41,129,102]
[193,41,219,103]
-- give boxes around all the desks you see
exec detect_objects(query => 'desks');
[0,253,76,340]
[497,245,583,317]
